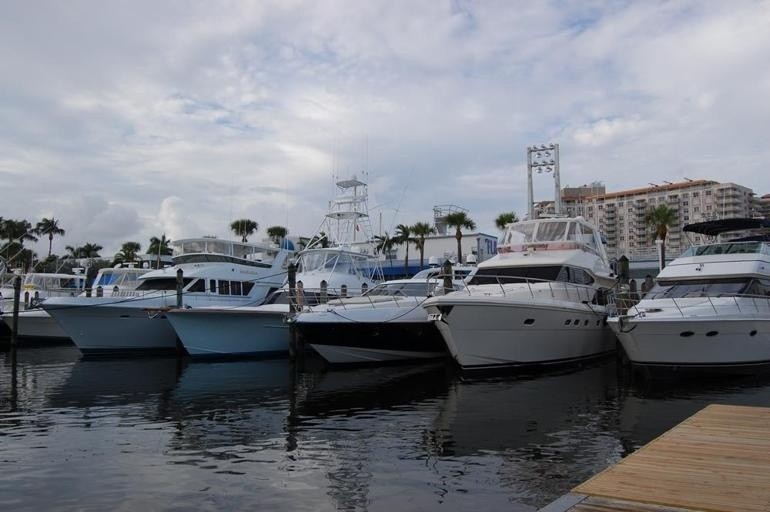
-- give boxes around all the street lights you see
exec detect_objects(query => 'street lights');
[525,143,560,219]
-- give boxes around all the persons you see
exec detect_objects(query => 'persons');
[466,250,477,263]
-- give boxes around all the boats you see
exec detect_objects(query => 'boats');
[39,238,292,361]
[606,192,770,385]
[56,348,173,401]
[431,364,619,456]
[422,216,648,381]
[0,258,110,314]
[163,165,390,366]
[281,254,474,372]
[0,255,37,298]
[167,352,289,409]
[0,253,174,349]
[288,356,445,422]
